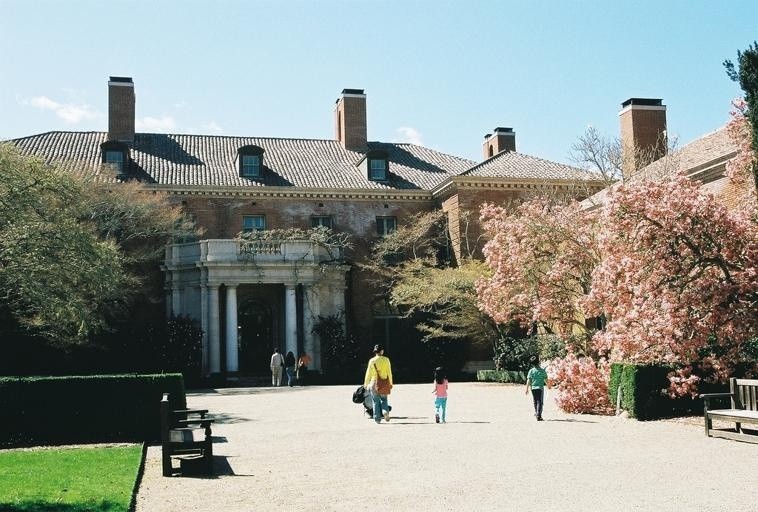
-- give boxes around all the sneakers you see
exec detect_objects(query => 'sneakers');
[436,415,440,423]
[535,415,543,421]
[382,410,390,421]
[376,419,380,424]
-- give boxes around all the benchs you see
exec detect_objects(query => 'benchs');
[699,377,758,444]
[159,392,216,478]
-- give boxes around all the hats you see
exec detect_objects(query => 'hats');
[432,367,443,371]
[373,344,382,352]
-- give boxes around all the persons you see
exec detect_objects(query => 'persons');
[364,344,392,424]
[526,355,551,421]
[432,367,448,423]
[363,383,391,419]
[269,348,285,387]
[299,352,312,386]
[285,352,295,385]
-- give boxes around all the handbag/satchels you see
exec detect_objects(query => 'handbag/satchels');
[377,378,391,394]
[353,386,366,403]
